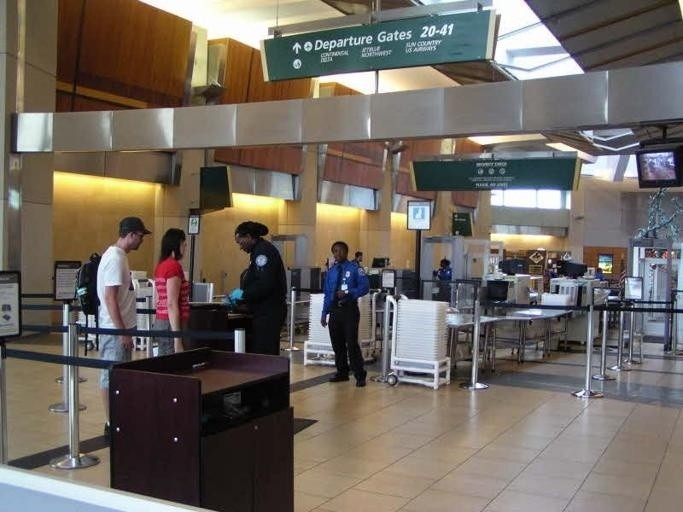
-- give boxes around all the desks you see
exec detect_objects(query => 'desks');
[284,293,574,376]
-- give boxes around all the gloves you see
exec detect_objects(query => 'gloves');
[224,288,244,306]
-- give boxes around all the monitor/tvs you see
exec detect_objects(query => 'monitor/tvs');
[372,257,389,268]
[635,145,683,188]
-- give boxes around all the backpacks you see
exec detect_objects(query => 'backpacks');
[75,252,101,315]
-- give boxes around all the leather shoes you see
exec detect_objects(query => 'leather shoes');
[329,374,366,386]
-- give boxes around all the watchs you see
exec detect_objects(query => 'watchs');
[345,289,349,295]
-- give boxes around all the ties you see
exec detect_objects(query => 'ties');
[333,266,342,298]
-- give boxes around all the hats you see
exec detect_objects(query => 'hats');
[119,217,152,233]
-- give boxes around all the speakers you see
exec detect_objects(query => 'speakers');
[200,165,232,209]
[452,213,472,236]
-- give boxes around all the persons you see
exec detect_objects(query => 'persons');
[594,268,604,282]
[432,257,452,299]
[153,227,191,357]
[320,241,370,387]
[91,215,150,435]
[350,250,364,266]
[233,221,289,356]
